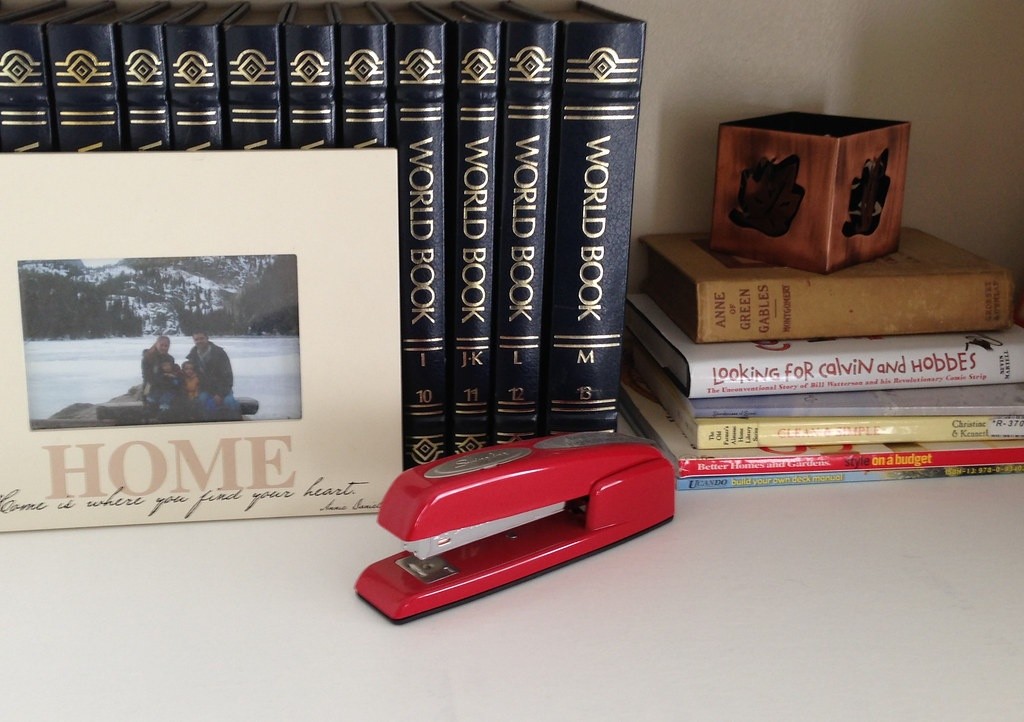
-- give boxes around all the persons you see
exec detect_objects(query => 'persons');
[185,328,243,421]
[141,336,184,423]
[155,357,179,412]
[177,361,200,414]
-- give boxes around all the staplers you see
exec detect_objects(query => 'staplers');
[354,429,676,627]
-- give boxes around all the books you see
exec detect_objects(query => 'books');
[1,1,647,477]
[619,224,1024,492]
